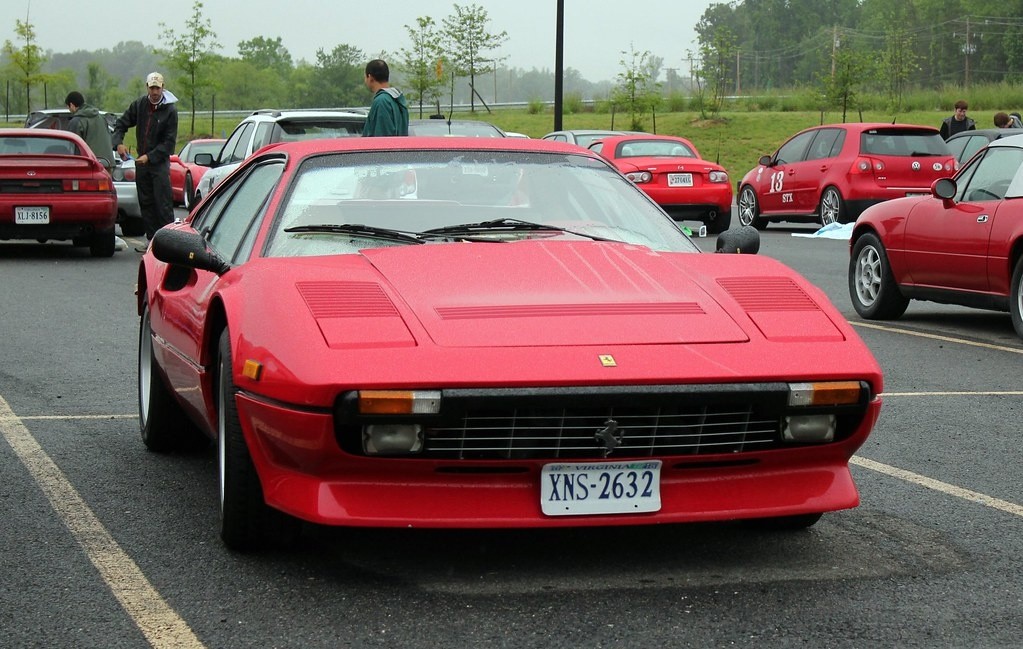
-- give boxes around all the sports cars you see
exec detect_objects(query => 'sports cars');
[167,138,228,210]
[135,135,885,555]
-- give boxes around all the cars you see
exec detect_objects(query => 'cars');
[845,134,1023,346]
[945,129,1023,170]
[584,134,735,236]
[539,128,651,149]
[409,114,535,183]
[0,127,118,259]
[735,120,959,231]
[17,108,147,239]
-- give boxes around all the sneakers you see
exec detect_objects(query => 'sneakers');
[135,240,150,251]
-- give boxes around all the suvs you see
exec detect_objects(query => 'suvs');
[186,108,420,215]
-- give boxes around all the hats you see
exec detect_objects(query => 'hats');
[145,72,164,88]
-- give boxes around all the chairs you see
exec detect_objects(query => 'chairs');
[44,145,70,155]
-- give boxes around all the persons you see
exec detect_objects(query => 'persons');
[994,112,1023,128]
[111,71,178,253]
[65,91,116,179]
[940,99,976,141]
[355,59,409,199]
